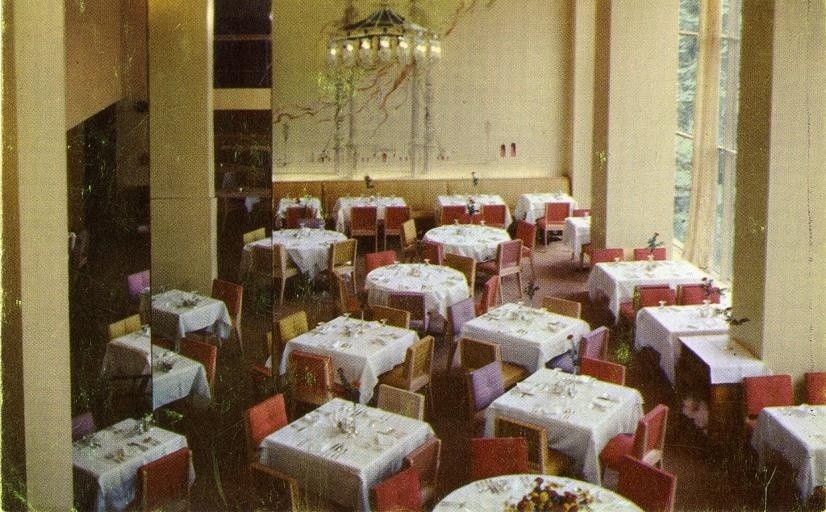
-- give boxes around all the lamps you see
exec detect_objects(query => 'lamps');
[326,0,442,66]
